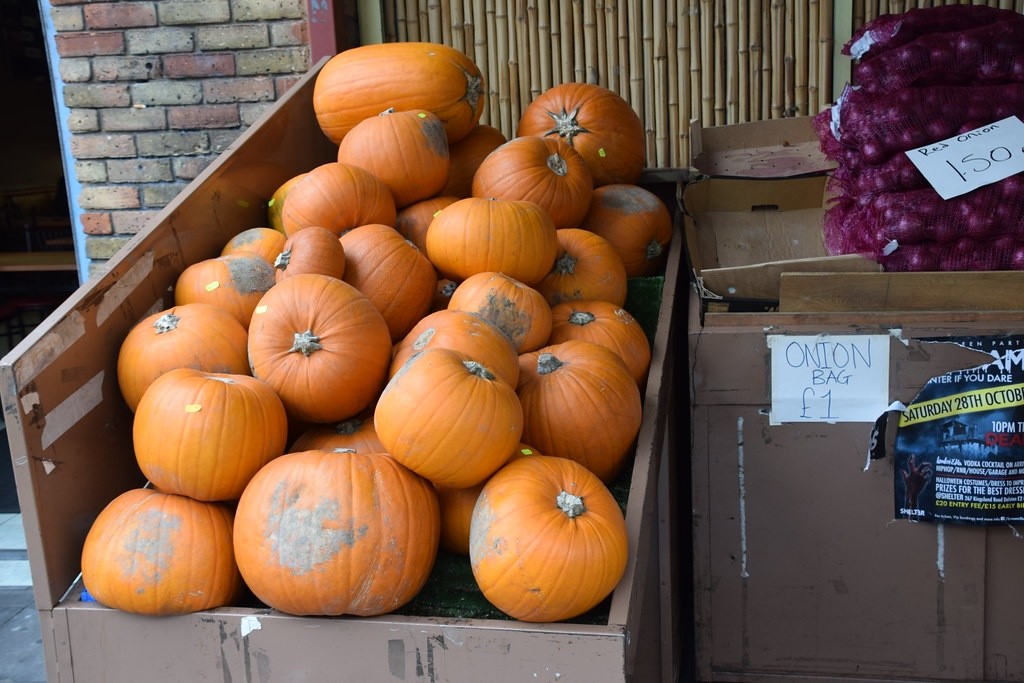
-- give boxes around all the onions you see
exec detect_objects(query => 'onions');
[830,3,1024,272]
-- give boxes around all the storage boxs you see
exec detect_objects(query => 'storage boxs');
[0,54,1024,682]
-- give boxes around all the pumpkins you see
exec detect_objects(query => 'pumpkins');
[77,42,674,625]
